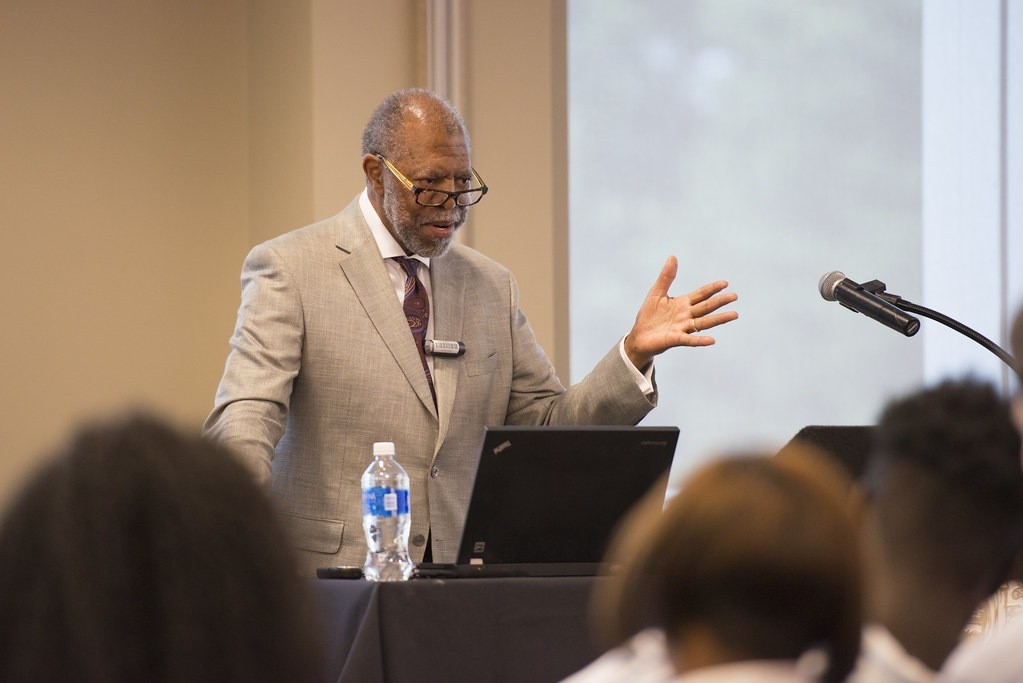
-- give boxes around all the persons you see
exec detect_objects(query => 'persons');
[559,296,1023,683]
[197,87,738,578]
[0,400,328,683]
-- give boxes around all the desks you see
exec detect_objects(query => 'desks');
[296,576,625,683]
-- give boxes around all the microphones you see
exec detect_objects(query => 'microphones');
[817,270,920,338]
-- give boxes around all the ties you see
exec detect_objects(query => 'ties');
[391,256,436,409]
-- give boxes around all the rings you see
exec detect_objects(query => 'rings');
[692,318,699,331]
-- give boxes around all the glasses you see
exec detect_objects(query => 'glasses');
[372,153,486,208]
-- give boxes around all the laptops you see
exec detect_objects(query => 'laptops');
[316,425,681,580]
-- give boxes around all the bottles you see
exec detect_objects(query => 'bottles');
[360,441,414,583]
